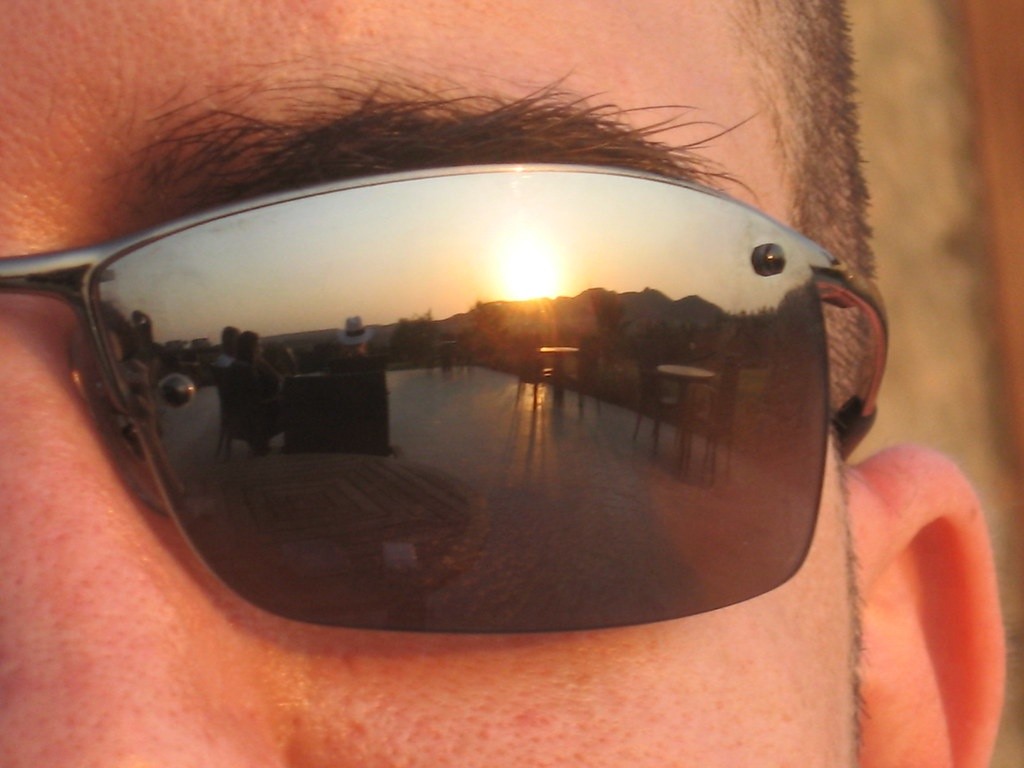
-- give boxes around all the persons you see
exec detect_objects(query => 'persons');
[1,0,1005,768]
[336,315,376,354]
[215,326,287,396]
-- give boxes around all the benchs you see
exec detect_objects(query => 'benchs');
[281,372,390,456]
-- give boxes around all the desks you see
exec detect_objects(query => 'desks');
[540,348,580,404]
[655,365,717,471]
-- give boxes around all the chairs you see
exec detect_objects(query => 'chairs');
[684,383,741,486]
[208,362,270,457]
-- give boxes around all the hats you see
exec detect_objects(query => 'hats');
[337,316,375,348]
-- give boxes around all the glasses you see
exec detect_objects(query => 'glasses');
[0,162,890,635]
